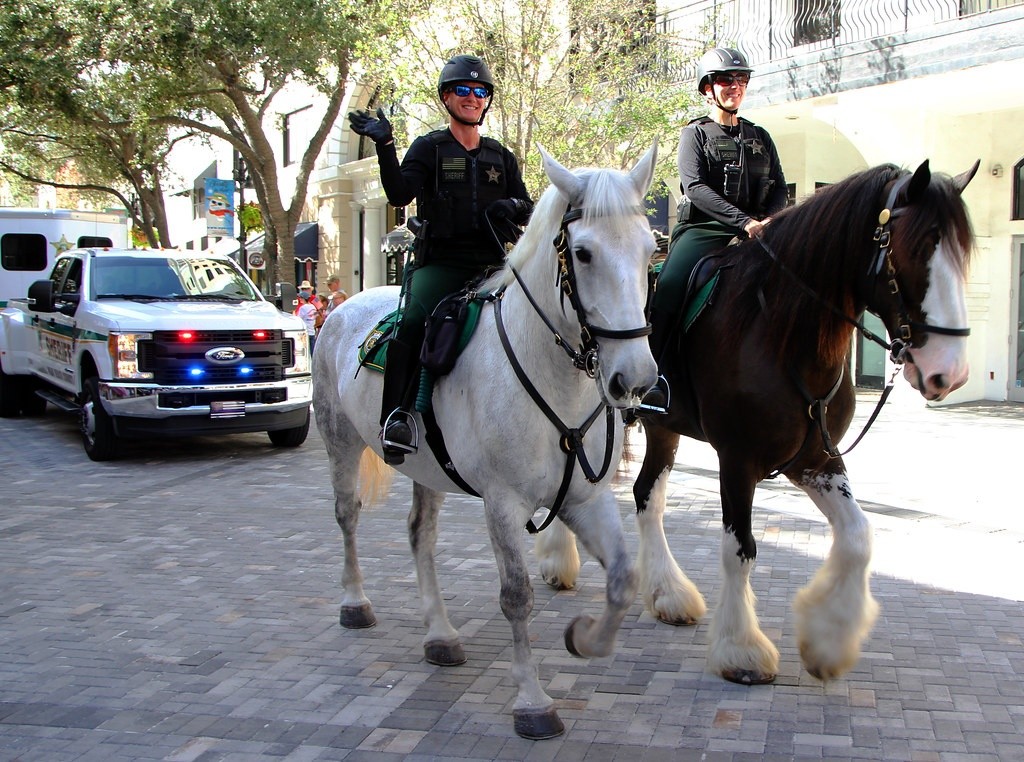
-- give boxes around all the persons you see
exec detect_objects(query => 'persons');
[632,47,789,418]
[295,275,349,356]
[349,55,533,465]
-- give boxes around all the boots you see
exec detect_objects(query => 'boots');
[654,302,691,399]
[378,338,422,465]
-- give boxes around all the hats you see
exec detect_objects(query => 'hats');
[323,275,340,284]
[298,281,314,291]
[297,292,310,299]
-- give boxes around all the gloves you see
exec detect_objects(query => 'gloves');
[490,199,515,226]
[348,107,393,145]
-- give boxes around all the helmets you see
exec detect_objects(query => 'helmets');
[437,55,494,129]
[697,48,755,115]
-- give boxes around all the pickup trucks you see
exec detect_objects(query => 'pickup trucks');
[0,246,315,462]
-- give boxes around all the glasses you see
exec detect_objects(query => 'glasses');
[712,73,749,87]
[447,84,487,98]
[301,288,309,290]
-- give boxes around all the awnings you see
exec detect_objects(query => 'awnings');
[207,226,265,256]
[380,221,417,253]
[236,222,318,263]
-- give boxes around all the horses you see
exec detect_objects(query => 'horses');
[308,140,664,743]
[535,155,983,689]
